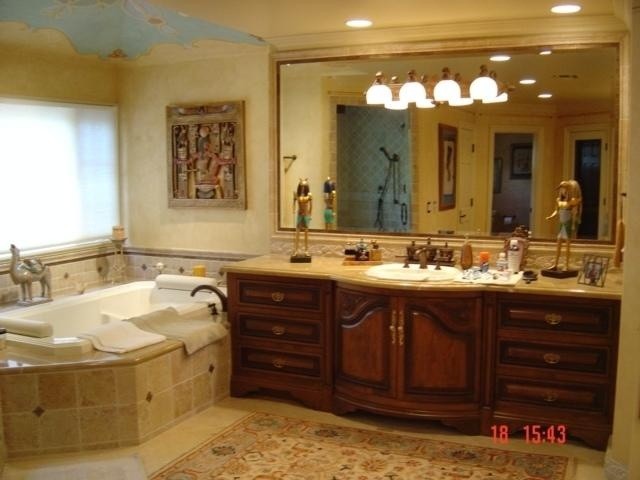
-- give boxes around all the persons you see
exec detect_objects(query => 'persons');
[588,265,598,277]
[323,177,336,230]
[547,180,576,272]
[292,176,313,257]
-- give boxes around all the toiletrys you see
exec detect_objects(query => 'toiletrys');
[476,228,481,236]
[344,239,382,261]
[496,252,507,273]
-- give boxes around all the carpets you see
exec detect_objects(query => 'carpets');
[143,411,580,480]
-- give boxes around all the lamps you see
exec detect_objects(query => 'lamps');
[399,68,428,103]
[382,76,409,111]
[482,70,509,105]
[363,71,393,105]
[448,73,474,108]
[413,75,436,111]
[468,64,498,100]
[432,66,461,102]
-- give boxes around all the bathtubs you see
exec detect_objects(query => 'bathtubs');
[0,275,224,355]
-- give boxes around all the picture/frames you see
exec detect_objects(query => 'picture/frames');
[436,122,459,214]
[163,99,248,212]
[509,141,531,181]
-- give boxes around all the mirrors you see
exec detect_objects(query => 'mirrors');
[275,40,621,247]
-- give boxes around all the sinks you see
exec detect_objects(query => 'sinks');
[364,263,461,282]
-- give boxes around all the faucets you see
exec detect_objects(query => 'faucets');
[190,284,227,312]
[438,229,454,235]
[415,241,433,269]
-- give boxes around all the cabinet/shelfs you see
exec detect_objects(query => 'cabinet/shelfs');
[328,282,487,436]
[225,272,332,413]
[479,289,621,453]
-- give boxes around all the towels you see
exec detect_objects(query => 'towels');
[75,321,166,354]
[129,306,228,356]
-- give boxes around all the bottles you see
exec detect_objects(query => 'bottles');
[357,245,369,260]
[496,252,507,271]
[371,245,381,261]
[507,240,521,274]
